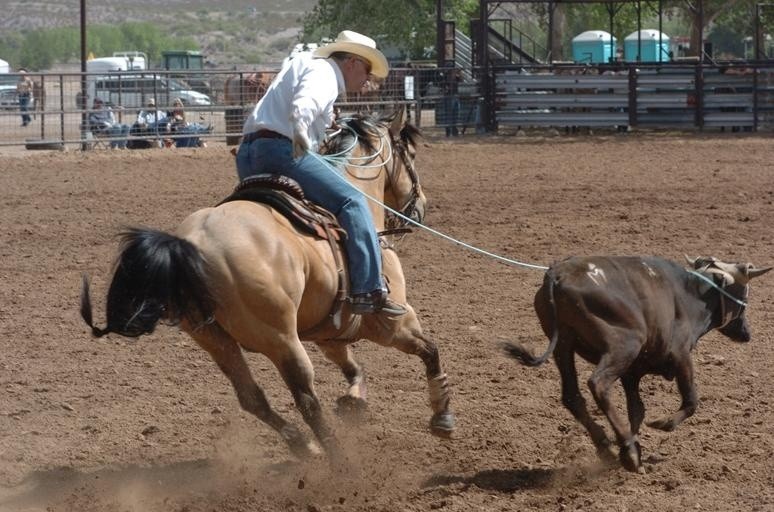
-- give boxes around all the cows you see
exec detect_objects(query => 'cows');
[496,252,774,476]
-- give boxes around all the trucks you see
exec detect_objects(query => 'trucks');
[1,59,37,114]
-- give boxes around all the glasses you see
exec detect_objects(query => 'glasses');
[348,56,371,74]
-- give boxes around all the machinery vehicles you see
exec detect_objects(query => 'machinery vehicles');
[152,47,220,104]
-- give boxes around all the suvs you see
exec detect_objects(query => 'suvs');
[74,75,212,111]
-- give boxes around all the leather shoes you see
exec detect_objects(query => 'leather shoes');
[352,292,407,317]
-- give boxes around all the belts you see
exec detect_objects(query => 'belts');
[242,130,288,139]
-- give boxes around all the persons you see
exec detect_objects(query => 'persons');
[234,30,409,318]
[79,96,131,151]
[13,68,34,130]
[434,60,466,136]
[169,97,213,145]
[139,97,167,127]
[126,114,167,147]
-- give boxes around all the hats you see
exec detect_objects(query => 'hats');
[94,97,103,103]
[142,98,154,105]
[312,30,389,78]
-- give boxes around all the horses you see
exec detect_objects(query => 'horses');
[345,74,381,110]
[224,71,270,145]
[78,101,454,462]
[388,63,449,125]
